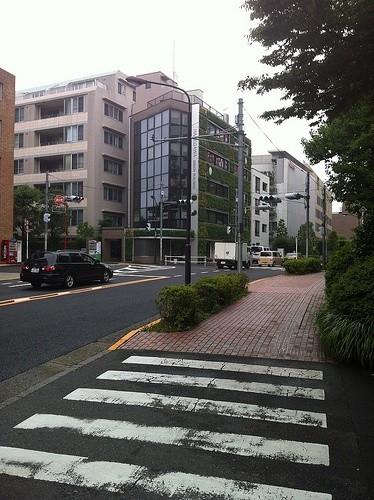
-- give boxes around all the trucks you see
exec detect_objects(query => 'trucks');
[250,245,270,264]
[214,242,253,269]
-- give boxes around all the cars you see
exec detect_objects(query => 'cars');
[284,253,304,261]
[19,249,113,288]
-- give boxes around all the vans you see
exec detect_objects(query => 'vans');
[258,251,283,267]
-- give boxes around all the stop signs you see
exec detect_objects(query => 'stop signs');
[54,195,65,205]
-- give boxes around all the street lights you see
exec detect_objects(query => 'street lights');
[127,76,193,287]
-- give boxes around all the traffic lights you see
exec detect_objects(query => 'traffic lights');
[66,195,84,203]
[259,196,281,203]
[176,199,187,205]
